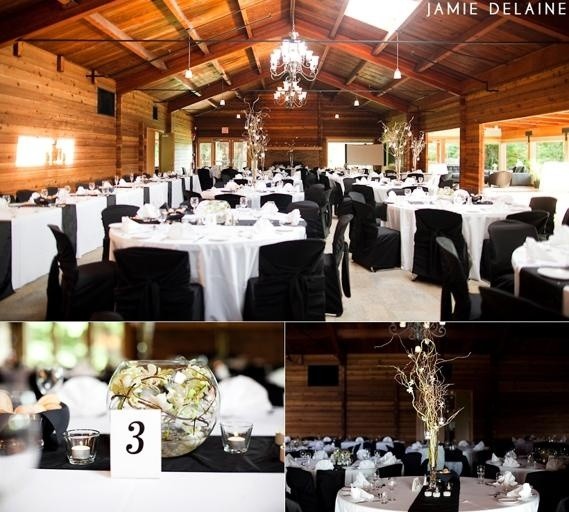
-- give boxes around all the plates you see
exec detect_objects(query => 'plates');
[537,267,569,279]
[497,497,519,504]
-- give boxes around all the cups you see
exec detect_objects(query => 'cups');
[496,473,505,489]
[305,453,311,465]
[130,173,134,184]
[40,188,48,198]
[219,421,254,454]
[240,197,248,212]
[404,189,411,201]
[477,465,485,481]
[3,195,11,204]
[61,429,100,465]
[371,470,379,488]
[89,182,95,192]
[190,197,199,214]
[300,450,306,460]
[386,477,398,501]
[114,175,119,188]
[156,209,167,227]
[380,489,388,504]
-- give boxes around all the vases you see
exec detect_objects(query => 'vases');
[289,153,294,167]
[428,433,440,492]
[413,155,417,170]
[395,156,402,180]
[251,160,258,188]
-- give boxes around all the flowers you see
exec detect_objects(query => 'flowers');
[393,340,471,430]
[242,96,271,160]
[288,141,295,152]
[411,131,426,154]
[378,116,416,156]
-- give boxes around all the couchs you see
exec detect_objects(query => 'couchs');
[488,171,513,186]
[511,166,531,186]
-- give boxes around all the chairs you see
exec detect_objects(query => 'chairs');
[378,463,404,477]
[412,208,472,285]
[213,194,244,205]
[488,219,538,290]
[75,181,88,191]
[320,174,329,189]
[434,236,480,322]
[259,193,292,206]
[46,187,57,195]
[291,201,320,220]
[529,197,557,236]
[113,244,205,322]
[322,211,353,316]
[244,238,327,321]
[365,441,376,457]
[404,452,422,475]
[473,449,499,479]
[479,283,560,322]
[16,188,34,202]
[305,183,324,206]
[353,443,362,461]
[47,222,115,320]
[235,178,249,184]
[343,177,355,194]
[197,167,213,190]
[280,178,294,186]
[372,164,381,173]
[316,470,344,501]
[350,183,386,221]
[439,179,454,189]
[506,209,550,234]
[286,466,315,505]
[221,168,238,178]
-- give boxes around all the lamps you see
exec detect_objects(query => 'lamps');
[394,31,401,80]
[388,322,447,339]
[354,72,360,107]
[270,32,319,109]
[185,30,193,80]
[218,74,228,108]
